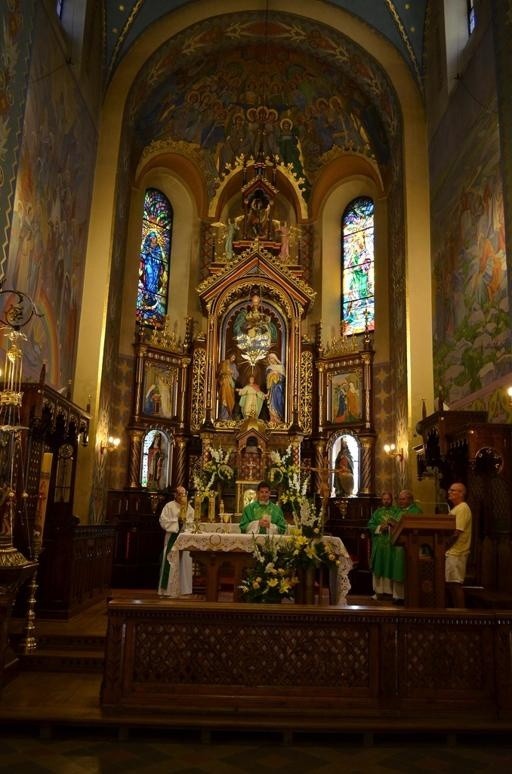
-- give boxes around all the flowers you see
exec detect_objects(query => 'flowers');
[191,442,341,603]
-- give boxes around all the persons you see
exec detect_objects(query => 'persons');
[335,438,354,497]
[264,353,286,423]
[368,492,397,600]
[158,376,172,418]
[140,233,165,319]
[336,383,347,417]
[147,433,164,492]
[388,489,423,600]
[445,483,472,608]
[239,481,286,534]
[236,375,269,419]
[215,352,239,421]
[346,382,360,417]
[155,65,366,203]
[158,486,195,600]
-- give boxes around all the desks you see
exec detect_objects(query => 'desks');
[178,532,342,609]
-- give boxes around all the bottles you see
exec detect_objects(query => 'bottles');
[85,394,92,414]
[65,378,73,402]
[38,358,48,384]
